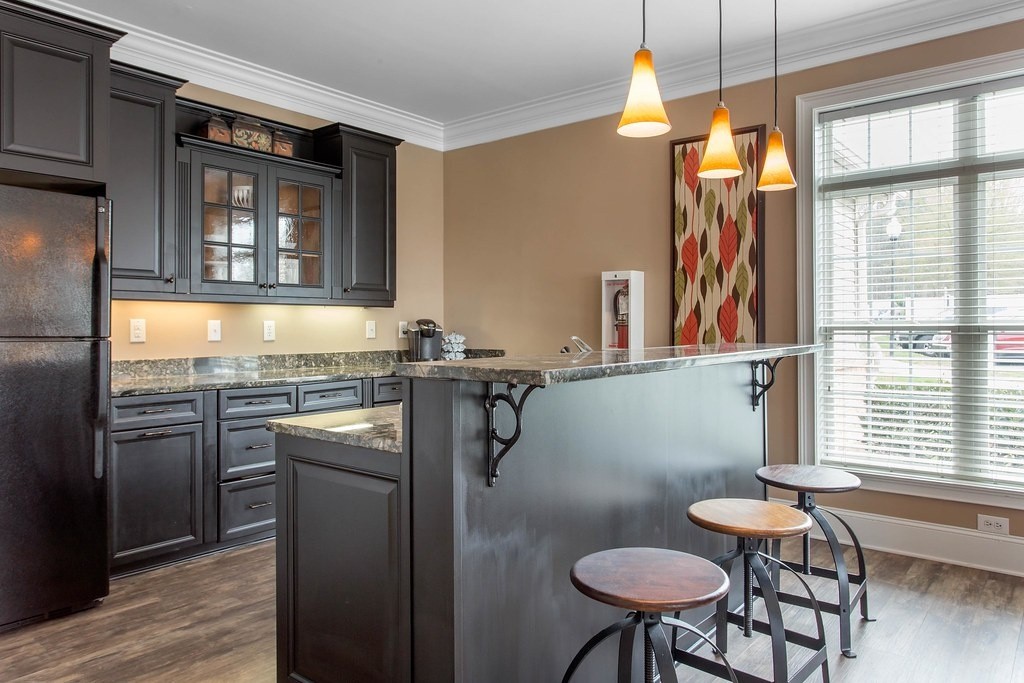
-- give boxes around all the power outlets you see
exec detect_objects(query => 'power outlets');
[263,320,276,341]
[977,513,1010,535]
[365,321,376,339]
[130,319,145,342]
[208,320,222,342]
[398,321,408,339]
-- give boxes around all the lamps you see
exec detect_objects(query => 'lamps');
[756,0,797,192]
[616,0,673,138]
[696,0,744,179]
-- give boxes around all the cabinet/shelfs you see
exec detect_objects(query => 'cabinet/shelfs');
[372,376,403,407]
[0,0,129,195]
[274,432,402,683]
[176,132,344,305]
[217,379,363,542]
[295,122,405,310]
[110,391,205,572]
[109,57,189,303]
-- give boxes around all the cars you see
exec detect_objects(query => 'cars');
[895,304,1024,361]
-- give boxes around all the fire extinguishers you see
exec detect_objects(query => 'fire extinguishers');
[614,285,628,349]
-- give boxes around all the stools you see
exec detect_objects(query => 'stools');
[561,546,739,683]
[739,463,878,659]
[670,497,830,683]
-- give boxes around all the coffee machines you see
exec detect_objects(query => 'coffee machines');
[407,320,443,363]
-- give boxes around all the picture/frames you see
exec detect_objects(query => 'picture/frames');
[669,124,767,347]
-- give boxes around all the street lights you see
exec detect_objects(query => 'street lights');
[884,216,902,358]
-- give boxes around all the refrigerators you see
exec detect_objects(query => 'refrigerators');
[0,184,114,638]
